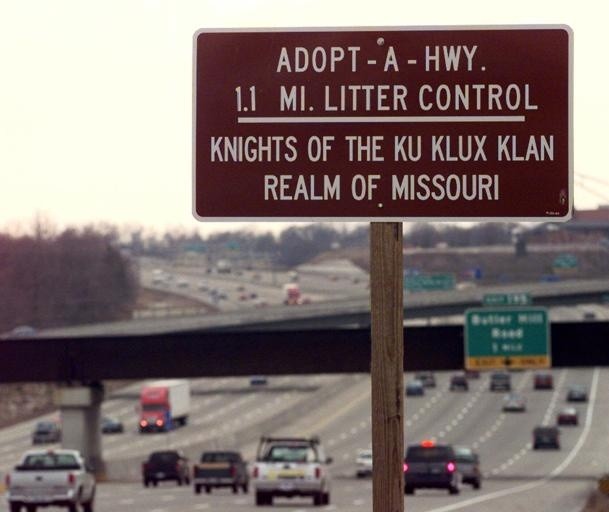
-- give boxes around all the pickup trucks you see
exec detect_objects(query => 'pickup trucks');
[141,435,332,506]
[4,446,96,512]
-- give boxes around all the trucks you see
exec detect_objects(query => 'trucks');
[135,379,191,432]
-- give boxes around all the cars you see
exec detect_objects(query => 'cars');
[405,370,552,413]
[403,439,482,495]
[355,451,373,477]
[101,415,123,433]
[533,385,588,449]
[32,420,62,443]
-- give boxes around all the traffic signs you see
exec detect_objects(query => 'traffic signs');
[465,308,551,370]
